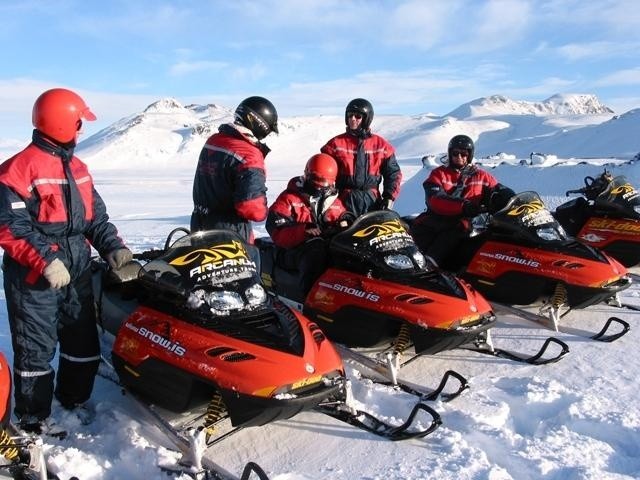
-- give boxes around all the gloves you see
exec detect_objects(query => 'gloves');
[42,257,71,290]
[105,245,134,270]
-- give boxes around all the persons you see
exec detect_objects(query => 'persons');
[266,154,347,286]
[0,88,134,436]
[319,98,403,218]
[0,342,52,480]
[423,136,515,251]
[190,96,279,249]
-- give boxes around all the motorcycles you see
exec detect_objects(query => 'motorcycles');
[245,203,572,405]
[401,186,639,346]
[548,164,639,267]
[71,221,446,480]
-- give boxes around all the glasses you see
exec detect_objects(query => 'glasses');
[346,113,362,119]
[451,150,468,157]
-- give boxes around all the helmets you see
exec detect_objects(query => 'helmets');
[448,134,475,168]
[344,98,374,131]
[305,152,340,192]
[32,88,97,145]
[234,95,280,140]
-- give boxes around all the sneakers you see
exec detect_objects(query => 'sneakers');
[73,404,93,426]
[21,421,68,441]
